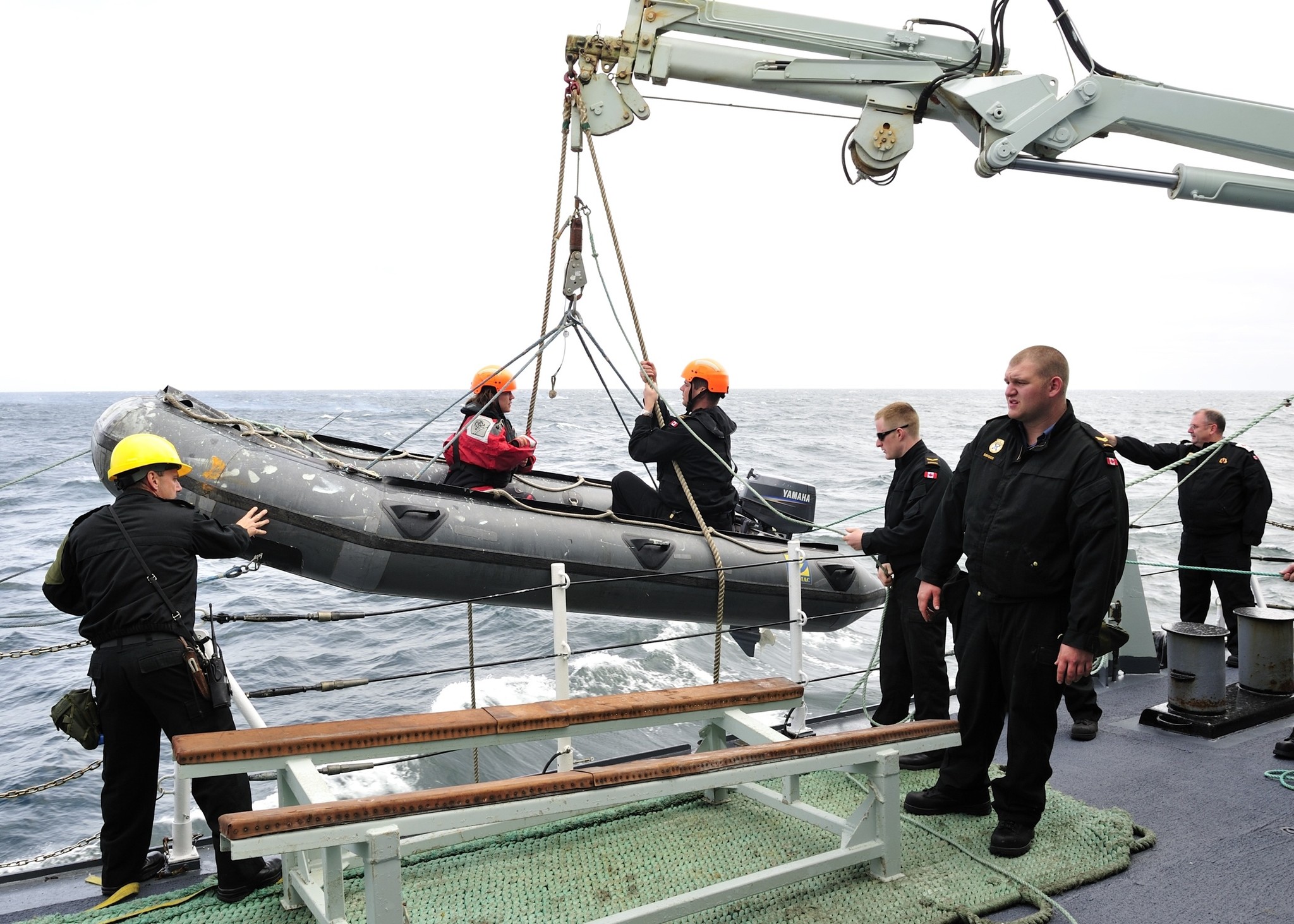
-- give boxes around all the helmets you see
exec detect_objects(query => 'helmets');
[471,365,517,394]
[108,433,193,482]
[680,358,729,393]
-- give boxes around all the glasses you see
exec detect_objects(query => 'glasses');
[1188,423,1213,430]
[876,424,909,441]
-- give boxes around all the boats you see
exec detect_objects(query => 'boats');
[92,385,892,632]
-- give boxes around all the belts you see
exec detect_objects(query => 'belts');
[94,633,179,649]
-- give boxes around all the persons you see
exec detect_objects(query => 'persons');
[900,344,1129,856]
[846,401,952,771]
[40,432,283,903]
[442,366,541,501]
[1279,564,1294,582]
[1102,408,1273,665]
[609,360,739,532]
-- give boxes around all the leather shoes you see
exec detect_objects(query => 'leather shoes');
[903,787,992,817]
[217,858,283,902]
[1273,733,1294,760]
[1071,718,1099,742]
[1226,656,1238,667]
[989,821,1036,857]
[898,753,941,770]
[101,851,165,896]
